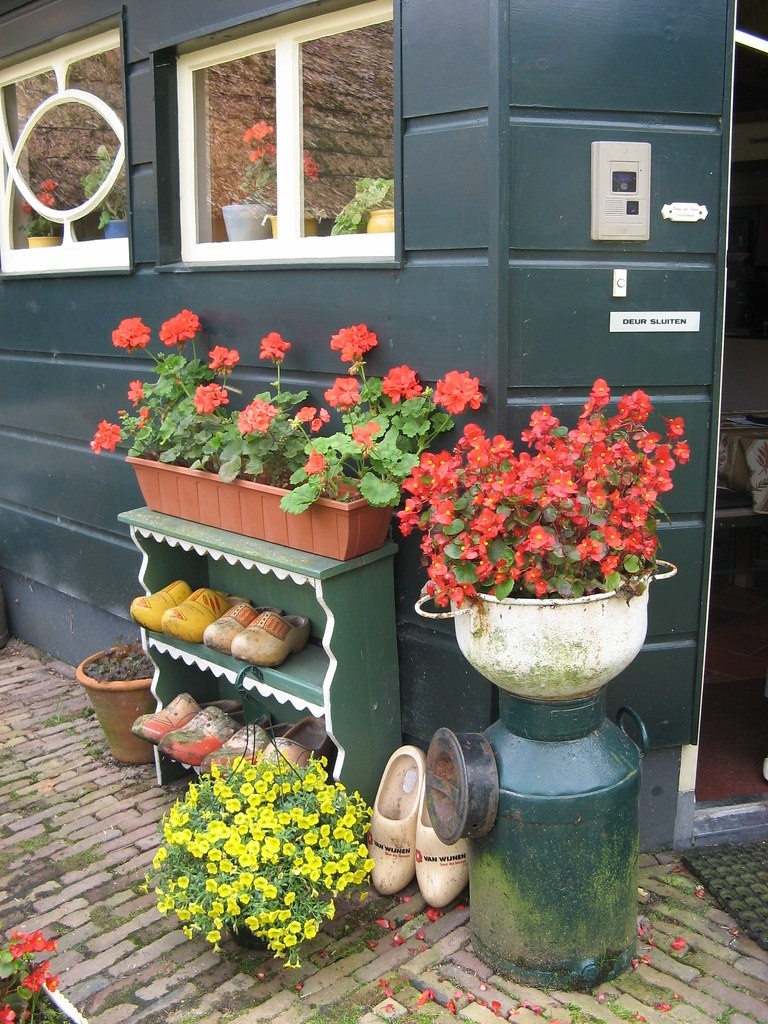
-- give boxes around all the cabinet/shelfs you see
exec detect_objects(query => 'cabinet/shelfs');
[116,507,402,808]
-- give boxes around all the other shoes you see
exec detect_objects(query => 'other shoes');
[131,578,229,635]
[366,742,425,896]
[131,692,244,747]
[203,602,285,656]
[231,610,310,667]
[416,764,469,908]
[160,587,251,645]
[255,715,332,787]
[156,705,269,767]
[200,722,298,778]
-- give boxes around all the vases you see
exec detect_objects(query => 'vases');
[414,559,677,699]
[270,215,317,237]
[221,203,275,241]
[42,980,90,1024]
[28,236,61,248]
[227,921,271,951]
[125,455,395,561]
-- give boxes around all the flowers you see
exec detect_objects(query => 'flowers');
[0,929,61,1024]
[90,309,485,516]
[235,123,277,205]
[303,150,319,185]
[17,178,64,237]
[137,752,376,970]
[395,378,690,615]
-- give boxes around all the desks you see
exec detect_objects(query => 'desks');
[716,409,768,591]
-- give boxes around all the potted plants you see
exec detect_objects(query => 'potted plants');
[80,145,130,238]
[330,176,394,235]
[75,633,158,764]
